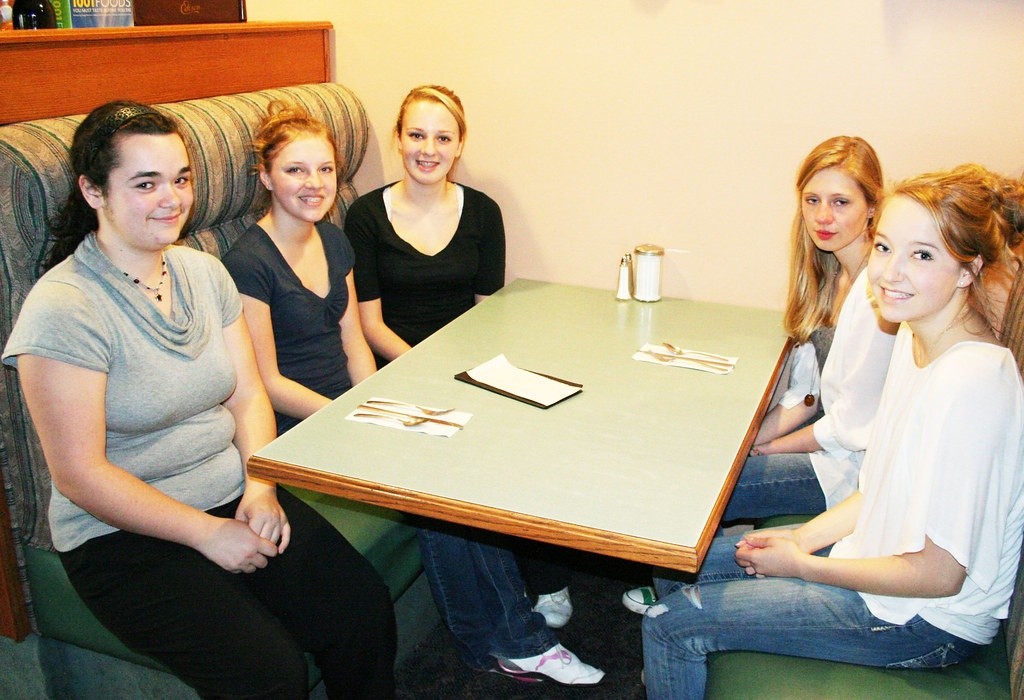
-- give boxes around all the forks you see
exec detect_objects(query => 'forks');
[367,401,455,416]
[354,414,431,426]
[648,350,727,371]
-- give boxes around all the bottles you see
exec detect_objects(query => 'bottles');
[616,252,633,300]
[12,0,58,31]
[633,244,665,302]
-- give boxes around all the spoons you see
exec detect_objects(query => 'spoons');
[663,342,728,362]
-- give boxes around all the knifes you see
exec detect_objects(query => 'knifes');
[357,405,464,429]
[637,350,735,369]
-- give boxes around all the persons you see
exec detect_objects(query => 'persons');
[622,136,898,626]
[1,101,397,699]
[221,100,606,692]
[641,165,1024,699]
[344,85,574,628]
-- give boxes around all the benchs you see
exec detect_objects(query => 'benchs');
[704,247,1024,700]
[0,83,431,700]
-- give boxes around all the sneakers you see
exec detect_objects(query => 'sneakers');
[622,586,658,615]
[475,644,605,687]
[533,586,573,628]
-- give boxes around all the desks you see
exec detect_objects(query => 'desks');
[245,280,793,586]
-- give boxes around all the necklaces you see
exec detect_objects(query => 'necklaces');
[803,254,872,407]
[111,252,168,302]
